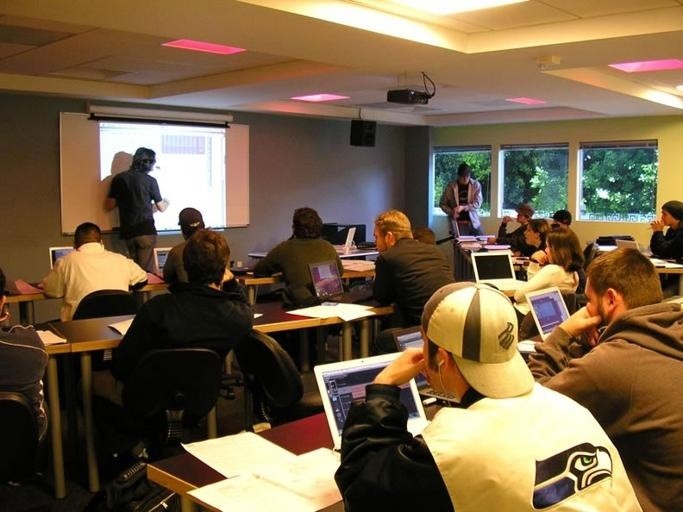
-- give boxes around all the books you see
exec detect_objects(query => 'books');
[454,233,496,248]
[33,328,67,347]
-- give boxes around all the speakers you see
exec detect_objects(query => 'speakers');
[350,120,377,146]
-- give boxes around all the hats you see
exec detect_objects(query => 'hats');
[549,210,572,225]
[660,200,683,221]
[178,207,205,232]
[421,281,536,399]
[515,204,535,217]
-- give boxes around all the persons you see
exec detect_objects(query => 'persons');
[647,199,682,298]
[0,269,48,489]
[162,205,205,283]
[252,205,344,306]
[524,246,682,511]
[437,160,489,253]
[497,203,585,332]
[38,221,148,324]
[104,145,167,272]
[73,225,253,489]
[412,224,436,244]
[369,207,457,355]
[331,279,643,512]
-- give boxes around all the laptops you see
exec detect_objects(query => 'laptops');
[332,227,356,250]
[307,259,371,303]
[616,240,638,250]
[391,325,460,405]
[153,246,173,278]
[471,252,527,291]
[314,352,432,451]
[49,246,73,270]
[525,286,571,341]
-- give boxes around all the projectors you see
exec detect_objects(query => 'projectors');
[387,89,428,105]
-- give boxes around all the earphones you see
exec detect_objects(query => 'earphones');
[439,360,444,366]
